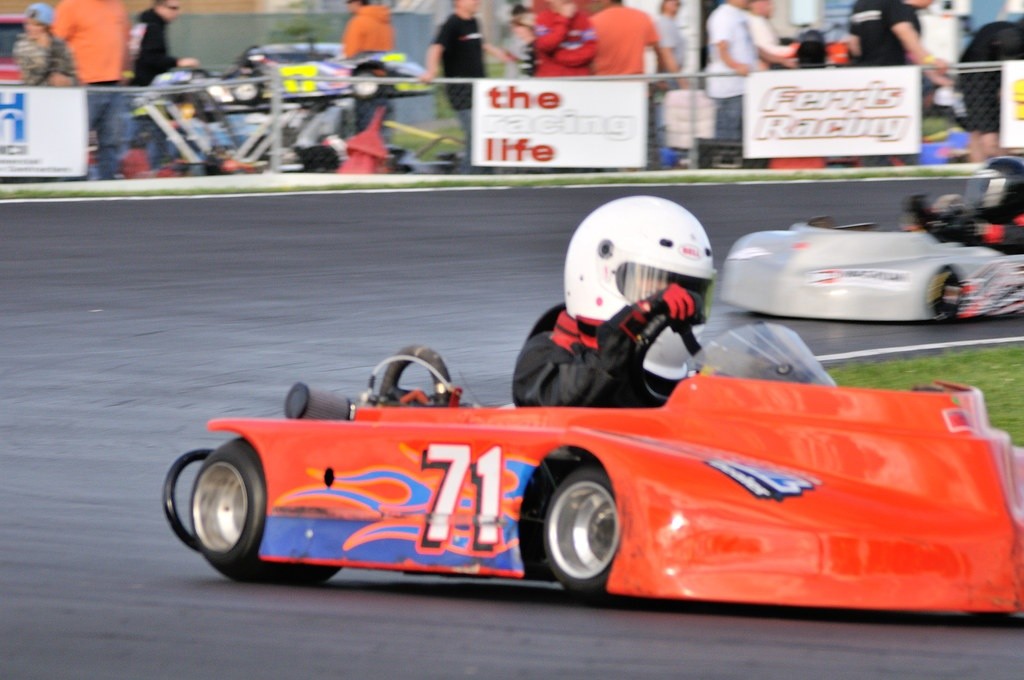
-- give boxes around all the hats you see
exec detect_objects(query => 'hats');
[25,3,54,25]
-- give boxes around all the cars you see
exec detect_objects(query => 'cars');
[1,13,456,177]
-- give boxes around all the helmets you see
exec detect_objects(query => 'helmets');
[963,155,1024,223]
[563,195,714,326]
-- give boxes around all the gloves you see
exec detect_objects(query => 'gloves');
[632,282,704,326]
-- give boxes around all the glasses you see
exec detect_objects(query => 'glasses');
[160,5,178,11]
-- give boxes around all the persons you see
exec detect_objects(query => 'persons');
[847,0,953,165]
[935,156,1024,252]
[344,0,396,60]
[706,1,799,140]
[422,0,514,174]
[512,194,812,408]
[16,3,78,87]
[119,0,198,173]
[502,0,687,168]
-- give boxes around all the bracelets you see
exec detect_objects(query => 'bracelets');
[924,55,936,63]
